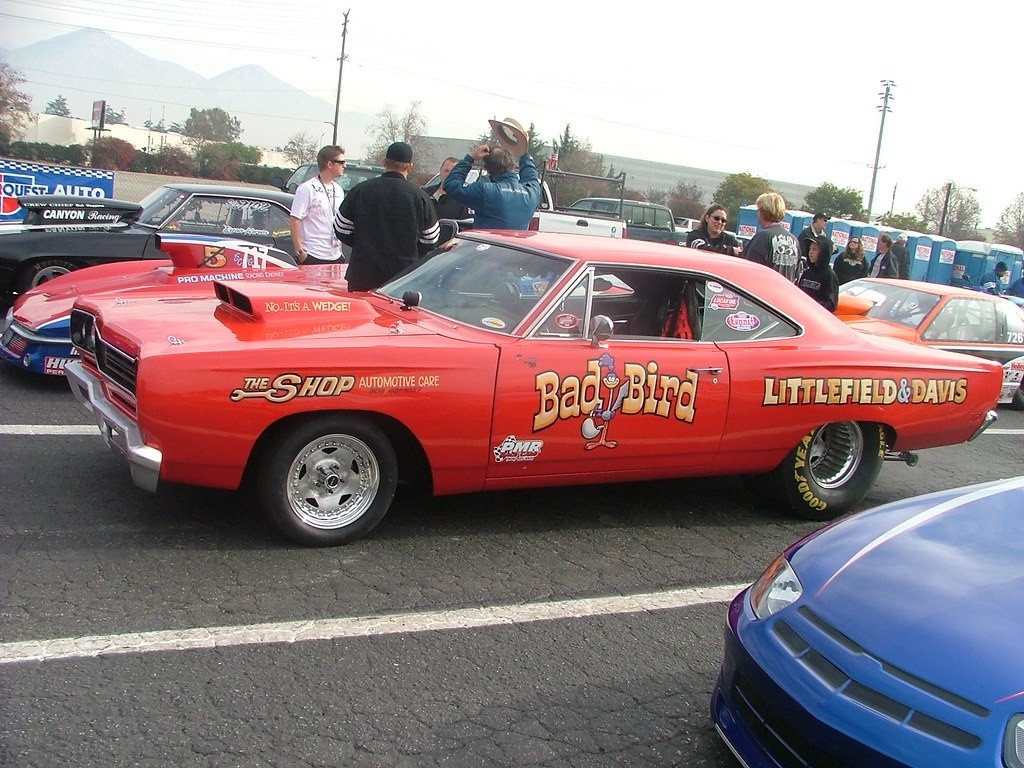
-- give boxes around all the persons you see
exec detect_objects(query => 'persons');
[333,141,440,292]
[443,132,541,295]
[417,157,476,252]
[797,235,839,315]
[738,192,802,286]
[869,233,899,279]
[289,145,346,265]
[1004,270,1024,299]
[798,212,838,269]
[833,236,869,286]
[687,204,744,256]
[891,234,910,280]
[978,262,1008,296]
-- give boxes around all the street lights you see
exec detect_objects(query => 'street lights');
[938,182,978,237]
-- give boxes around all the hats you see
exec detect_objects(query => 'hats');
[386,141,413,163]
[804,238,817,243]
[897,233,908,242]
[994,262,1007,272]
[814,212,831,221]
[488,117,529,156]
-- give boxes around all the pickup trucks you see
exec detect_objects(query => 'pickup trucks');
[419,168,628,239]
[564,197,689,249]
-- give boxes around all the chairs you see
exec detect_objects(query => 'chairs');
[248,209,265,232]
[225,206,243,227]
[627,278,701,339]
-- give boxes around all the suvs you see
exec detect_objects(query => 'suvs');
[271,162,387,206]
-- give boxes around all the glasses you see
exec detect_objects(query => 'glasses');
[820,218,827,223]
[331,159,345,166]
[850,241,858,245]
[706,214,727,224]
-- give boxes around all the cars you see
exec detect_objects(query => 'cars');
[0,182,356,322]
[62,230,1005,552]
[710,476,1023,768]
[832,277,1024,413]
[722,231,753,254]
[0,231,350,380]
[673,216,702,233]
